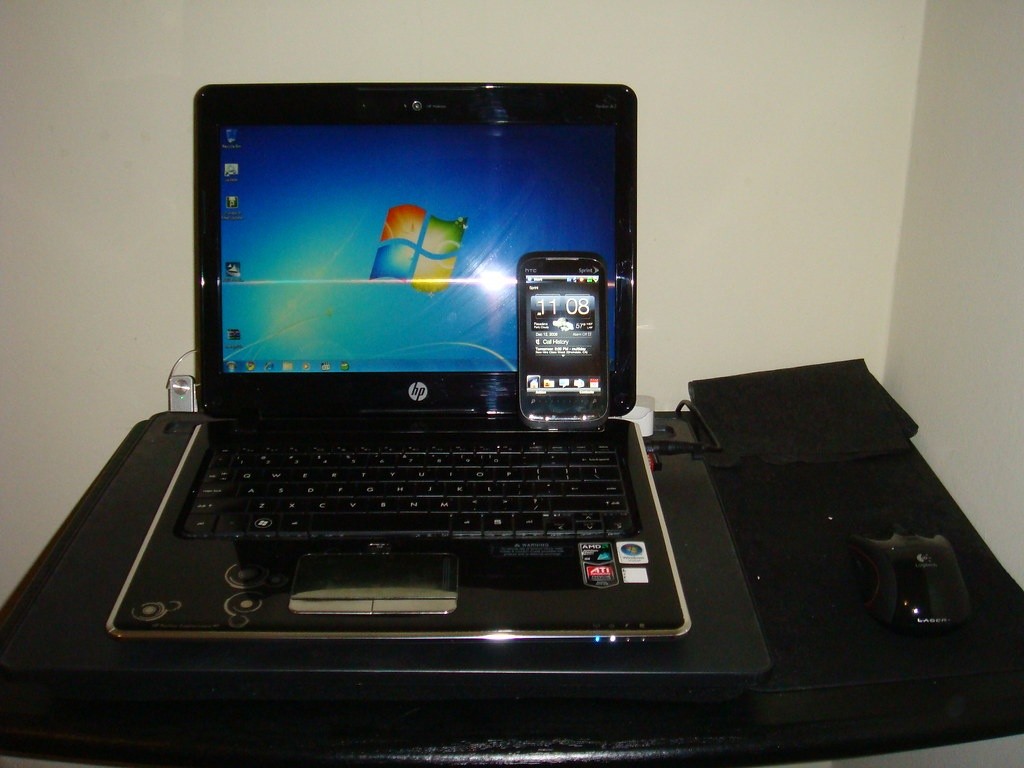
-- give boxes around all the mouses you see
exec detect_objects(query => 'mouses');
[847,523,970,640]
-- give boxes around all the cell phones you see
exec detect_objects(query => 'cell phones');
[515,251,609,430]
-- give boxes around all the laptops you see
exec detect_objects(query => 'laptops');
[106,84,692,646]
